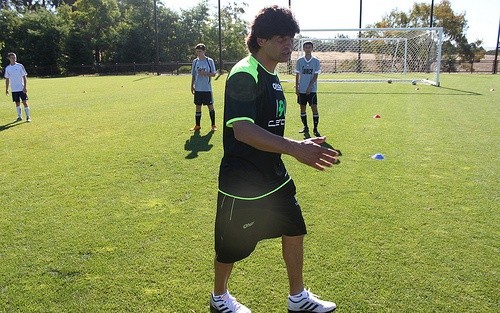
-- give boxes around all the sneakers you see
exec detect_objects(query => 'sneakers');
[210,291,251,313]
[287,288,336,313]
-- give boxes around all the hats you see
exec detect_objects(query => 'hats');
[196,43,206,50]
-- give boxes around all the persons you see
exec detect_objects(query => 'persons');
[209,5,338,313]
[296,41,321,136]
[4,53,32,122]
[189,43,217,131]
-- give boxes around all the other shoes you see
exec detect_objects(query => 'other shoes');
[211,125,218,131]
[26,118,31,122]
[17,117,22,120]
[313,128,320,136]
[299,127,309,133]
[190,125,200,131]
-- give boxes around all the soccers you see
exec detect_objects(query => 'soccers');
[411,79,417,86]
[387,79,393,84]
